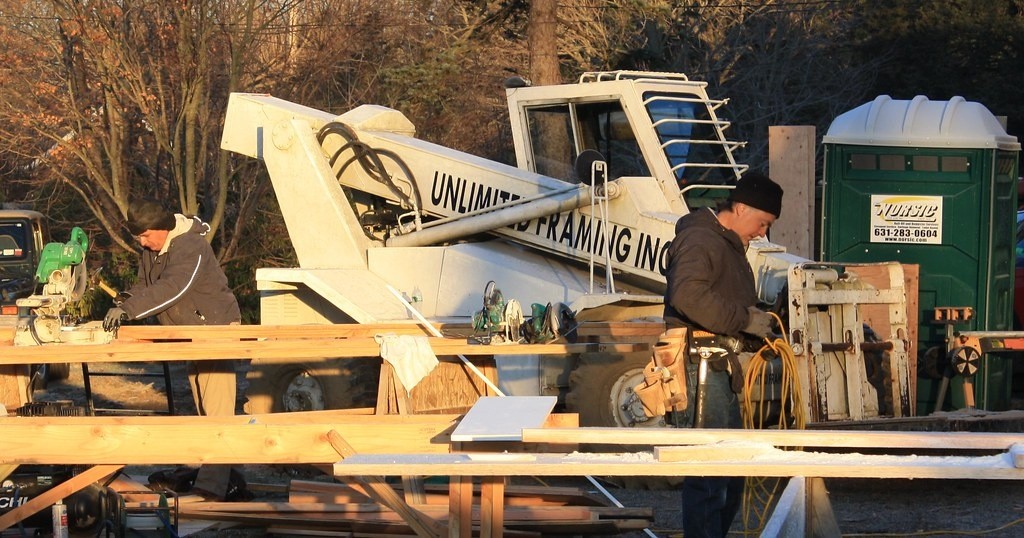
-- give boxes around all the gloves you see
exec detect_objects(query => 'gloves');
[741,306,772,338]
[112,293,133,307]
[102,307,126,332]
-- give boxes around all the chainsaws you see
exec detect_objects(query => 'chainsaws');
[16,227,90,316]
[471,280,524,342]
[519,301,580,343]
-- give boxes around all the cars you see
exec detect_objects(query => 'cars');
[1,209,71,389]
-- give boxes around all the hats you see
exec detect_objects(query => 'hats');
[727,171,784,219]
[127,200,176,236]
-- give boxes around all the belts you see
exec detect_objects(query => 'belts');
[692,331,715,338]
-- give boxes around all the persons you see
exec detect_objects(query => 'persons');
[662,170,783,538]
[101,196,248,501]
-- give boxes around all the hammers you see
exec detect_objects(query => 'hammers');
[689,346,726,428]
[90,266,125,305]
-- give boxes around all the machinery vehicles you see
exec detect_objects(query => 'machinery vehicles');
[218,67,851,490]
